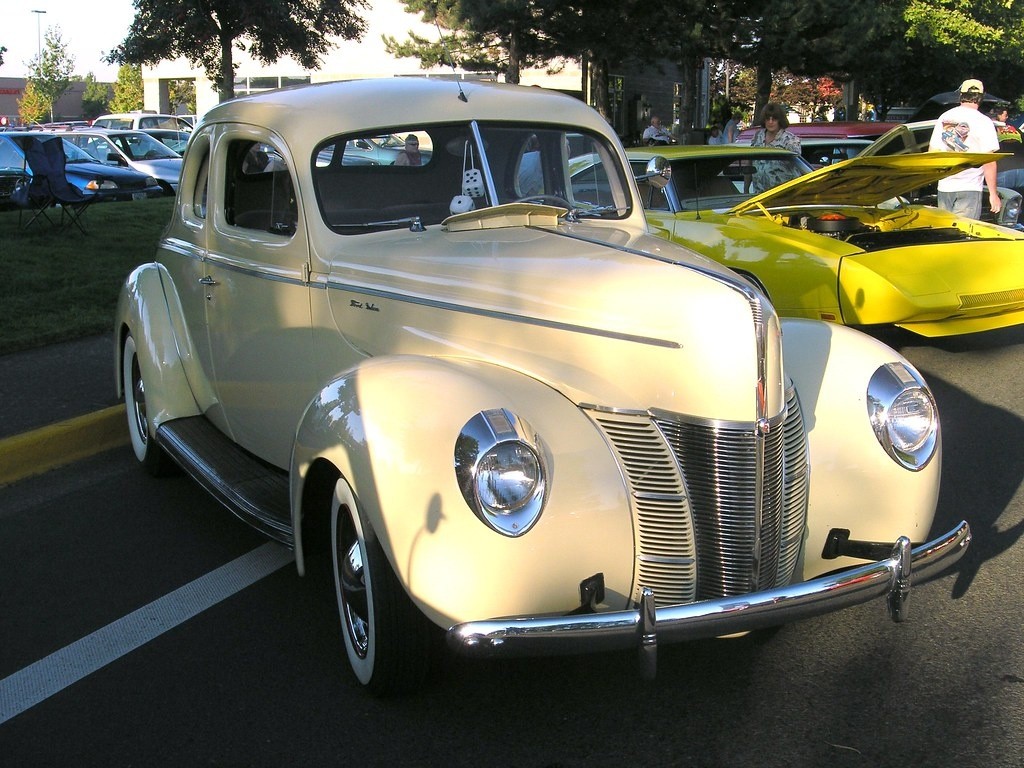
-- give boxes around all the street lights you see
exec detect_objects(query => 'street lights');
[32,10,47,78]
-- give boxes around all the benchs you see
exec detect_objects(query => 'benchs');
[233,201,451,237]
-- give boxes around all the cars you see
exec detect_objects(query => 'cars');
[540,144,1024,349]
[43,132,185,195]
[113,77,973,697]
[1,133,164,210]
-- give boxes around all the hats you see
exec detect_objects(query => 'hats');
[954,78,984,93]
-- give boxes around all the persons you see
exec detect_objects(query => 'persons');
[723,112,744,143]
[742,102,802,195]
[243,142,269,174]
[994,107,1009,122]
[928,78,1003,220]
[643,115,677,144]
[515,136,571,199]
[394,133,422,167]
[709,127,723,145]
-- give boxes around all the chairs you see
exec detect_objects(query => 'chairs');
[15,134,96,236]
[636,181,667,209]
[572,189,615,209]
[698,176,740,198]
[111,143,122,152]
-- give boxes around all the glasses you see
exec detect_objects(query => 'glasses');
[408,142,419,146]
[765,117,777,121]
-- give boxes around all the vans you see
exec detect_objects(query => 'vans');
[92,114,193,131]
[734,121,902,170]
